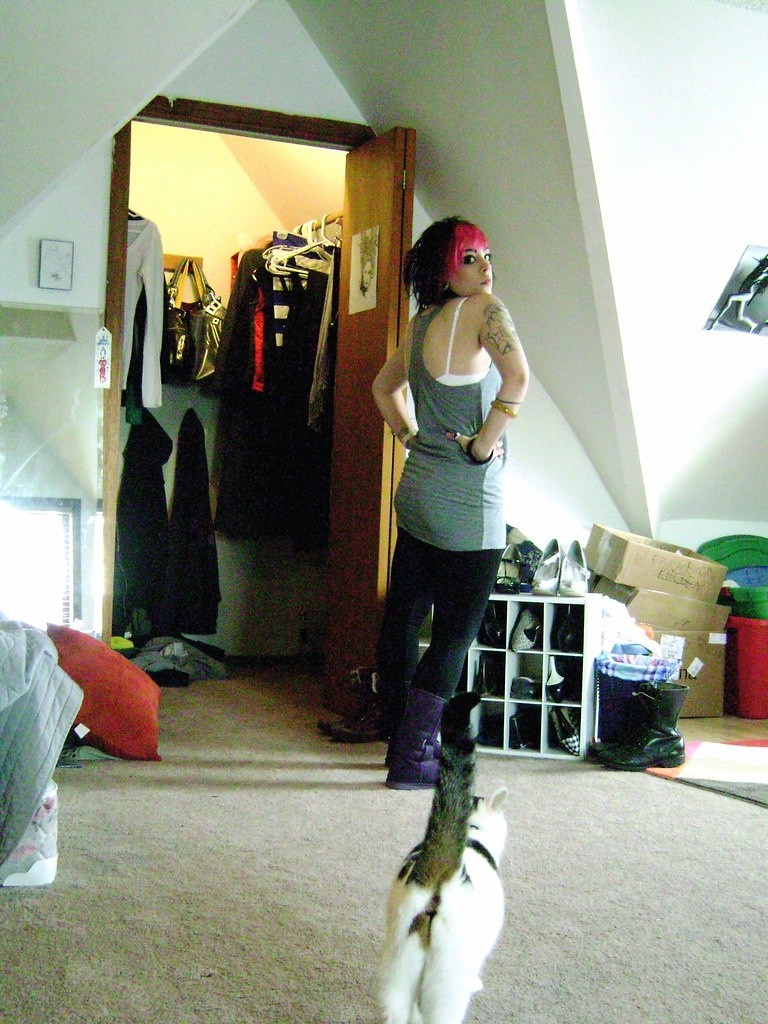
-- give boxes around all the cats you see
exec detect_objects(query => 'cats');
[373,692,508,1024]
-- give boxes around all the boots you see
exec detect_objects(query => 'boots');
[587,681,689,771]
[510,710,536,748]
[316,679,411,744]
[474,657,500,697]
[384,687,447,790]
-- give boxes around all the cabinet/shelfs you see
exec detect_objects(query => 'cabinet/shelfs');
[419,594,603,761]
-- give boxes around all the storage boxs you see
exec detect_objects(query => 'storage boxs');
[585,524,767,718]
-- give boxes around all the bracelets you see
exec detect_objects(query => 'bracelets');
[401,430,418,448]
[488,398,518,420]
[466,437,494,465]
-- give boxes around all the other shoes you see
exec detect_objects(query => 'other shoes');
[551,603,584,652]
[512,608,540,650]
[477,602,505,648]
[546,655,579,702]
[551,708,579,756]
[512,676,542,695]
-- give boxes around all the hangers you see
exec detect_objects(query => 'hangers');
[262,213,335,279]
[128,209,146,233]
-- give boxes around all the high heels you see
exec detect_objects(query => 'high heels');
[494,543,523,593]
[560,539,590,595]
[531,539,563,596]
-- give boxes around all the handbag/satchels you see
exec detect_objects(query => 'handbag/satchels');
[161,259,227,386]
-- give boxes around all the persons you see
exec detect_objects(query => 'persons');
[372,216,527,791]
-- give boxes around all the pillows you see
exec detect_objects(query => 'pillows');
[46,622,162,762]
